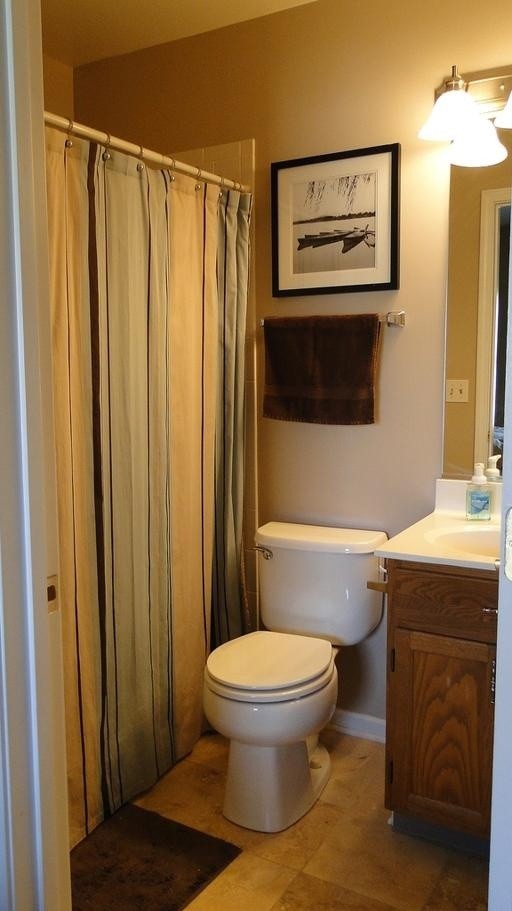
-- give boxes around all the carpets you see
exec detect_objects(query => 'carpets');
[70,801,243,911]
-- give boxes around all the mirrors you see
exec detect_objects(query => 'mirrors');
[474,185,512,480]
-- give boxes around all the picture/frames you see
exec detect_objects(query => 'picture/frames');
[269,143,400,298]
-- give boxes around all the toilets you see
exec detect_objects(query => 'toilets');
[202,520,389,833]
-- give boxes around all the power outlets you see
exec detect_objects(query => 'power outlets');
[445,380,469,402]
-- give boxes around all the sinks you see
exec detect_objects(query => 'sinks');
[423,524,502,560]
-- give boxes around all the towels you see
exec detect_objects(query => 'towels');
[261,312,379,425]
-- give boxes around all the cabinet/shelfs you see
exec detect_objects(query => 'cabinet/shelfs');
[384,559,499,857]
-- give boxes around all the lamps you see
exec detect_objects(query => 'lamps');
[417,62,506,167]
